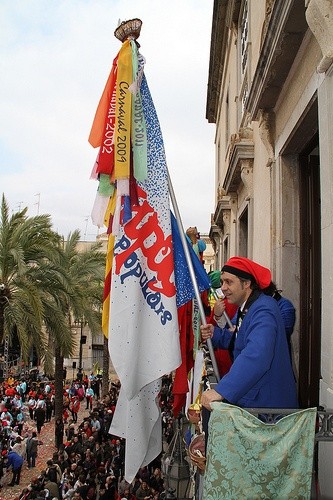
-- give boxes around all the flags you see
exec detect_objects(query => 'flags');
[87,36,212,486]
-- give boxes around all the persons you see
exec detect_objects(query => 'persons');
[212,278,296,384]
[198,272,239,380]
[0,366,180,500]
[198,256,300,423]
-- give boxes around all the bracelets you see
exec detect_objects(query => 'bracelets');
[213,307,224,317]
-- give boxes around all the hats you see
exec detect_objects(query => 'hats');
[220,256,272,290]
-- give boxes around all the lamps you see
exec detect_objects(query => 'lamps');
[161,413,191,500]
